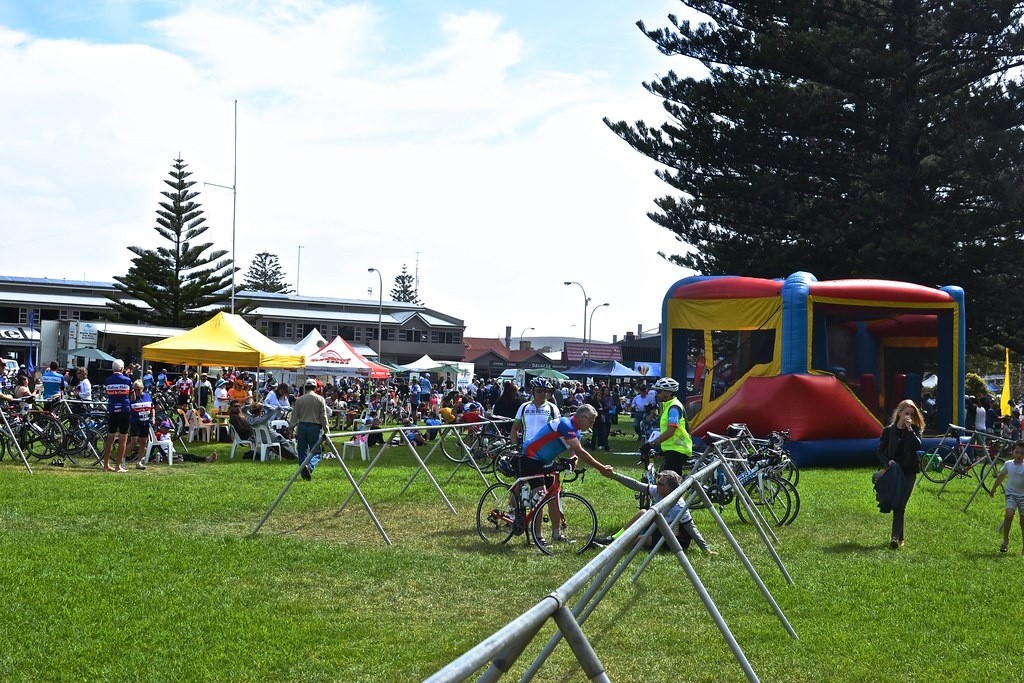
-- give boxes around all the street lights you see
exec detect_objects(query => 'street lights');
[588,303,610,360]
[368,268,383,363]
[521,327,534,341]
[563,282,591,358]
[296,244,305,296]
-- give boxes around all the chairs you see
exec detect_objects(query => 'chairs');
[342,423,372,463]
[190,408,218,441]
[144,423,175,465]
[251,424,284,464]
[228,422,255,463]
[351,408,372,431]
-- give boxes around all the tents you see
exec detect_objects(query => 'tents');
[139,311,477,404]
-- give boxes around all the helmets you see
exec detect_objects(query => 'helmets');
[530,376,553,387]
[651,377,679,392]
[498,457,517,478]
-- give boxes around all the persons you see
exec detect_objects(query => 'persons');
[0,356,93,456]
[511,375,720,558]
[103,359,155,472]
[493,376,662,453]
[879,399,926,549]
[990,440,1024,556]
[289,377,330,481]
[965,395,1024,462]
[94,340,493,467]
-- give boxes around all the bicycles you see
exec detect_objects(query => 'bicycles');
[683,422,800,528]
[0,384,186,468]
[609,428,636,438]
[439,419,523,486]
[476,455,598,558]
[634,447,655,511]
[919,428,1008,496]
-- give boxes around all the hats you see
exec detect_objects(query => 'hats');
[163,422,171,428]
[305,379,317,387]
[215,378,228,387]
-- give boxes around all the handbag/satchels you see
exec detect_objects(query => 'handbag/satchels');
[601,415,605,423]
[872,471,883,484]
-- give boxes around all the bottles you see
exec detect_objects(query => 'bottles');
[528,490,542,508]
[488,441,501,449]
[483,437,488,451]
[522,485,530,507]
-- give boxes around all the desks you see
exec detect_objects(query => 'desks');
[328,409,349,431]
[263,420,290,460]
[216,414,245,442]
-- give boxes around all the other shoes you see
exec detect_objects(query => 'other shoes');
[1000,544,1008,552]
[302,465,311,480]
[537,537,553,548]
[49,459,65,467]
[209,452,217,461]
[122,460,146,469]
[592,535,614,548]
[890,538,898,550]
[551,535,577,543]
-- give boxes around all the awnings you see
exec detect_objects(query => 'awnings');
[499,358,649,379]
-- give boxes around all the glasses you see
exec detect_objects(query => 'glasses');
[584,416,595,424]
[535,389,548,394]
[657,482,668,486]
[134,388,141,392]
